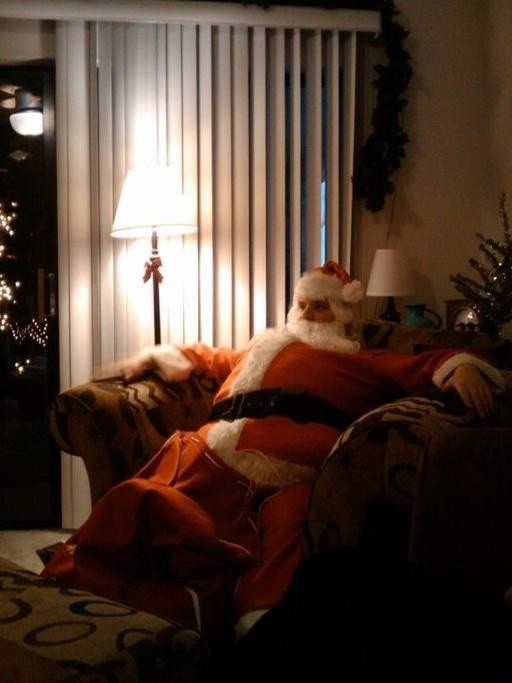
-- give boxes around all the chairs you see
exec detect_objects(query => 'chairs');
[52,318,511,678]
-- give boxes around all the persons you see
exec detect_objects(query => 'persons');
[40,259,510,648]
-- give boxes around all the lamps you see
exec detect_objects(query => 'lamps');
[109,165,199,349]
[364,248,420,324]
